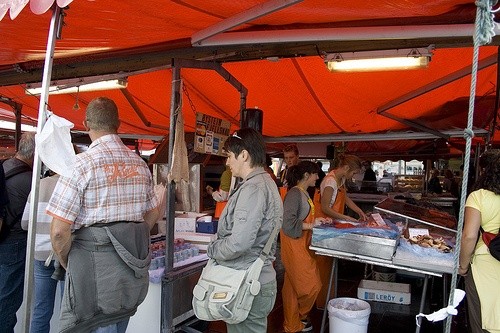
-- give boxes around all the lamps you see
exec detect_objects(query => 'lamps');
[324,47,434,71]
[25,73,128,97]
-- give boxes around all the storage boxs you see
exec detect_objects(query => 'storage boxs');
[174,212,207,233]
[357,279,411,305]
[196,221,218,234]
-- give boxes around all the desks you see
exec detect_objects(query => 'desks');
[314,252,447,333]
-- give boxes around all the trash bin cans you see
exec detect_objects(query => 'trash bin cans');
[327,297,371,332]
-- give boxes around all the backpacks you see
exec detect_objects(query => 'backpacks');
[0,159,32,244]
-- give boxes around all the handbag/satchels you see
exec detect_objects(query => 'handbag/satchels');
[191,253,266,325]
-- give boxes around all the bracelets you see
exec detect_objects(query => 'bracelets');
[458,264,463,270]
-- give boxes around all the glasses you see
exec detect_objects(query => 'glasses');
[229,132,242,140]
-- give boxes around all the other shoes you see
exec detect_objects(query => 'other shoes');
[316,305,329,310]
[300,319,308,325]
[299,324,312,331]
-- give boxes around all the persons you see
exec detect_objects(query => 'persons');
[458,151,500,333]
[277,143,298,200]
[0,130,80,333]
[282,159,333,333]
[427,160,476,199]
[206,158,243,218]
[45,97,159,333]
[362,160,377,192]
[262,152,279,185]
[314,155,370,314]
[204,126,284,333]
[316,161,326,187]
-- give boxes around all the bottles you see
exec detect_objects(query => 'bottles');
[148,238,200,270]
[413,166,424,175]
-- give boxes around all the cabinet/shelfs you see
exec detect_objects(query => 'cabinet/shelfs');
[393,175,425,192]
